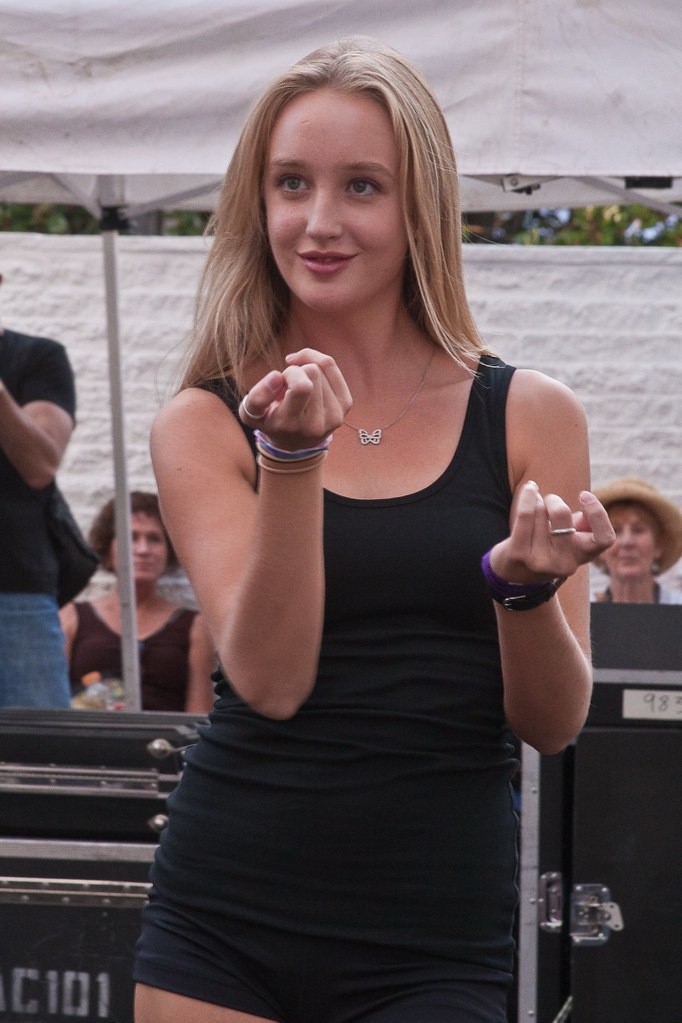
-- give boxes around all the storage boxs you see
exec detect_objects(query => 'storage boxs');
[516,604,681,1022]
[0,709,211,1023]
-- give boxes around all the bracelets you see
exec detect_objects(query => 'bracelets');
[480,550,568,611]
[253,428,333,475]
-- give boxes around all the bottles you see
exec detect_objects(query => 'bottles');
[82,670,124,715]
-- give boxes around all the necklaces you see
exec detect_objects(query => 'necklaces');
[344,350,435,446]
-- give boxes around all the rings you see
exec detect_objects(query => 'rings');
[244,396,269,421]
[553,528,576,534]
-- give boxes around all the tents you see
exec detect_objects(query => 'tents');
[0,3,681,696]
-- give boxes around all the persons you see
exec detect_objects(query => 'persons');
[0,271,100,712]
[60,490,211,714]
[593,475,681,607]
[134,34,618,1023]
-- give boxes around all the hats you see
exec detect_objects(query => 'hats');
[589,479,682,578]
